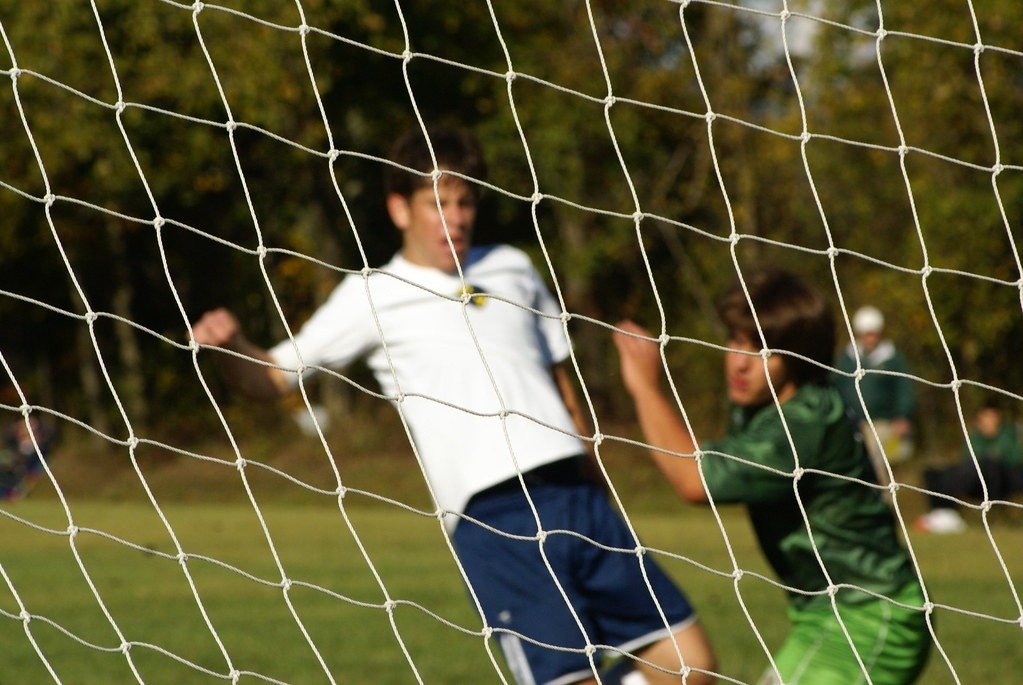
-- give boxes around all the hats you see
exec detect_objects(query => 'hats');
[852,306,884,336]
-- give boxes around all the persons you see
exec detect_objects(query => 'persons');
[918,400,1023,534]
[184,126,716,684]
[833,307,912,502]
[616,269,934,685]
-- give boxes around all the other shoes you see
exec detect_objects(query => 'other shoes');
[917,507,962,534]
